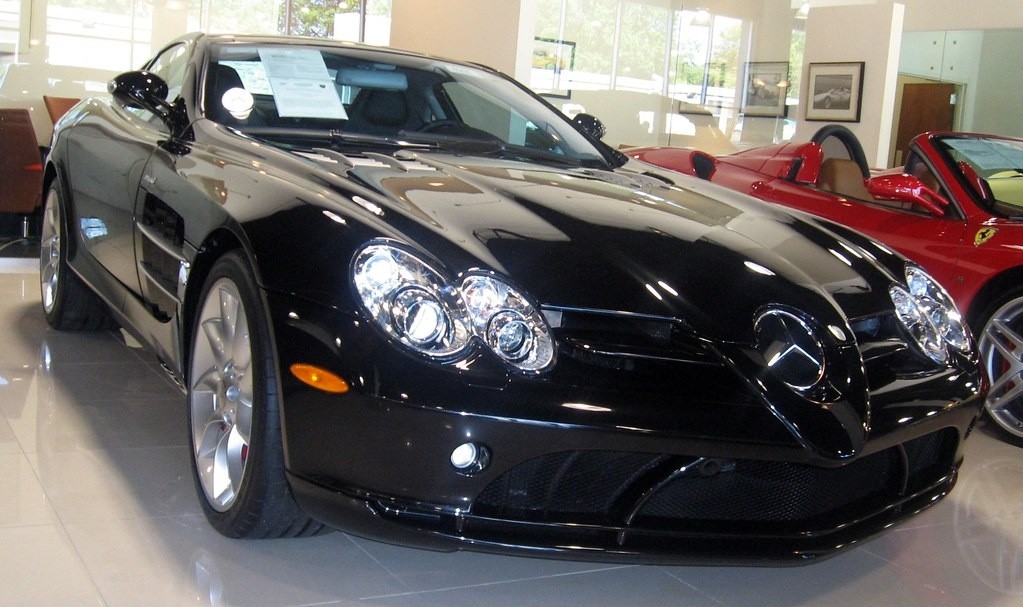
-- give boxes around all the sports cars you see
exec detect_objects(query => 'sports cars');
[33,32,985,568]
[611,123,1023,446]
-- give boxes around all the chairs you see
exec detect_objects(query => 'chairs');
[819,159,894,208]
[344,86,436,134]
[211,65,268,130]
[0,107,47,253]
[901,163,941,214]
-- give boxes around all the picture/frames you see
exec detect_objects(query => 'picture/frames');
[804,61,866,123]
[531,37,577,98]
[738,62,788,117]
